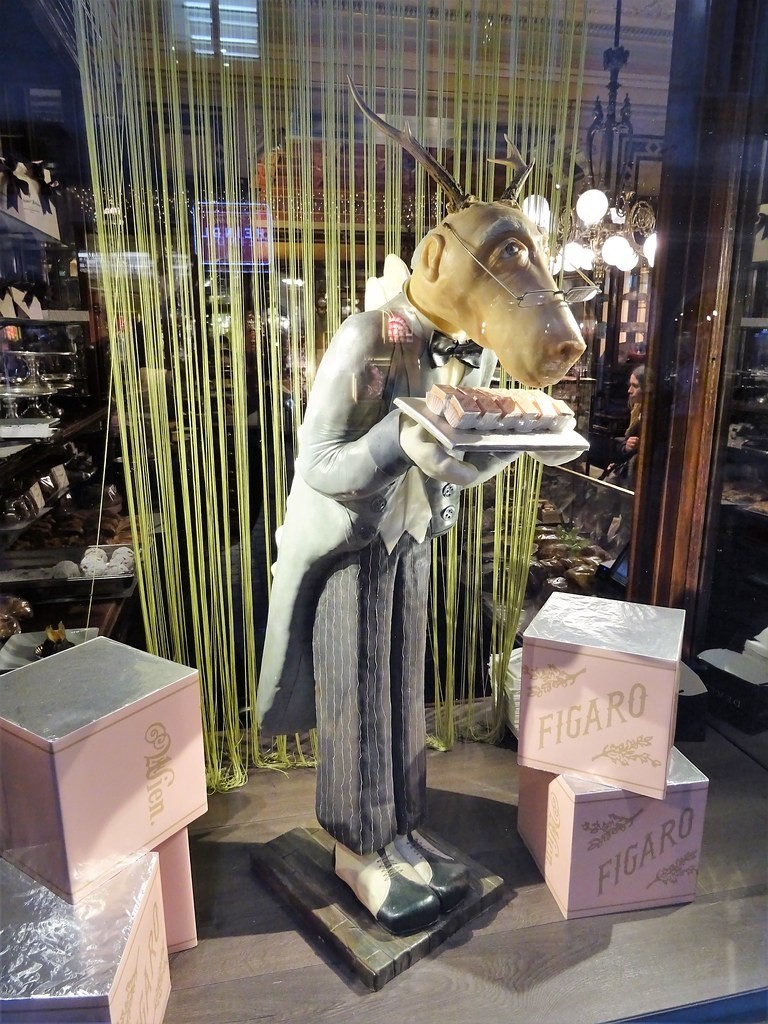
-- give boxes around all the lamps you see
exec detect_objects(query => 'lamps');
[523,0,657,274]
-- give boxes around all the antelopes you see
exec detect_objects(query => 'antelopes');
[343,72,587,389]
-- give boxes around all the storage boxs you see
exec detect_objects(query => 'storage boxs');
[518,744,711,919]
[0,635,210,1024]
[675,659,709,741]
[514,592,687,799]
[695,648,768,736]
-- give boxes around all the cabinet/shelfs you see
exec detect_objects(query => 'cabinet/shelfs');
[0,208,112,478]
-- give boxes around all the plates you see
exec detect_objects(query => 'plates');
[0,627,99,670]
